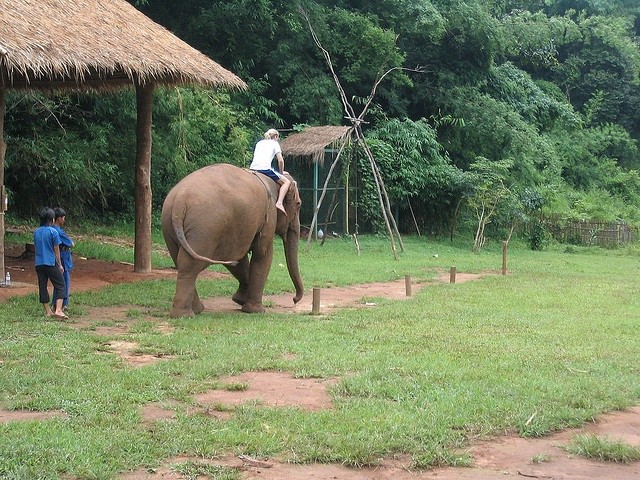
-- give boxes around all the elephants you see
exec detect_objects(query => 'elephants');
[161,163,304,319]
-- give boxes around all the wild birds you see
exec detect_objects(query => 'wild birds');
[332,231,341,238]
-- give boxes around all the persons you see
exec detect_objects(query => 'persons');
[52,207,75,314]
[33,207,70,319]
[249,129,292,217]
